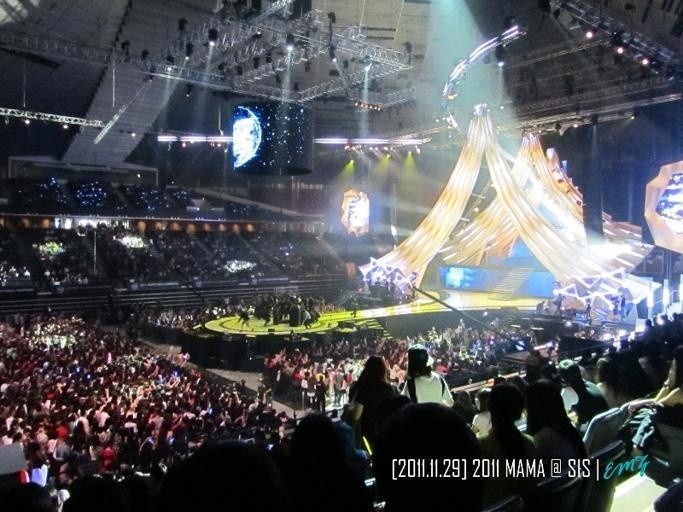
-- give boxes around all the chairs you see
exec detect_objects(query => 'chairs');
[0,184,683,511]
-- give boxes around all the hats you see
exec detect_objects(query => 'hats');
[408,344,428,362]
[560,360,581,382]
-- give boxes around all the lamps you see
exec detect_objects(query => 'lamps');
[0,0,683,173]
